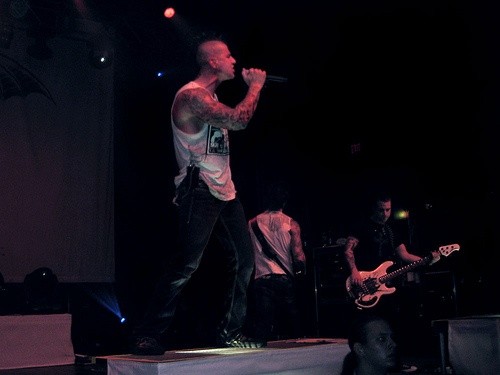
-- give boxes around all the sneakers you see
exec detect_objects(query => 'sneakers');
[395,360,417,372]
[135,336,165,355]
[217,329,267,348]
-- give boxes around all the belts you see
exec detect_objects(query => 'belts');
[197,180,208,189]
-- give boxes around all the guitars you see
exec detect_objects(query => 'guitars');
[346,244,460,308]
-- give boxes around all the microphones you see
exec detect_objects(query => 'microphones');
[266,75,288,82]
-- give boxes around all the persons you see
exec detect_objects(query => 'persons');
[343,195,441,374]
[247,188,308,346]
[129,39,268,357]
[346,312,398,374]
